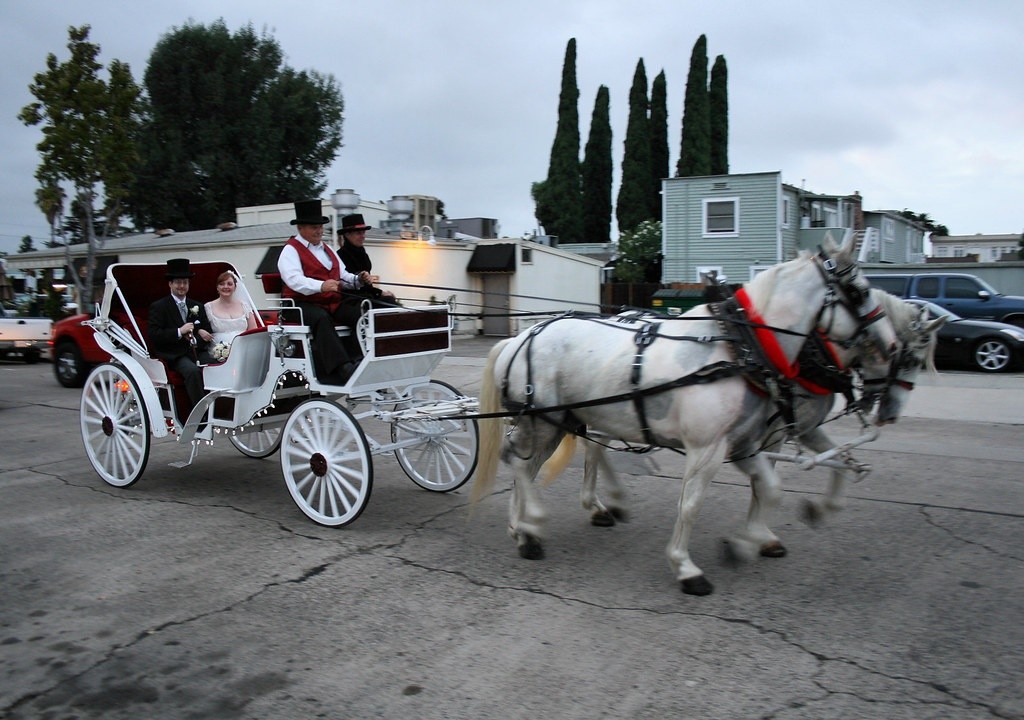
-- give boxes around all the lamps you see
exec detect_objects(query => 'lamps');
[417,226,436,245]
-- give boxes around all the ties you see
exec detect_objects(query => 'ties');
[180,303,187,324]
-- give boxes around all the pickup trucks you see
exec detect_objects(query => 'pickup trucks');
[1,301,56,365]
[50,307,290,390]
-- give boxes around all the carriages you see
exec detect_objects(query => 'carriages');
[78,224,949,599]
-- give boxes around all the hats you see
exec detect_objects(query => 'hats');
[163,259,196,281]
[290,199,330,226]
[336,213,371,234]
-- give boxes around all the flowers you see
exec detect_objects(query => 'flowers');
[188,306,198,324]
[213,341,230,363]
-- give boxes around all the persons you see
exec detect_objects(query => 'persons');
[276,199,374,381]
[146,258,260,425]
[333,213,398,310]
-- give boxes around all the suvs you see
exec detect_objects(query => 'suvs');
[860,271,1024,334]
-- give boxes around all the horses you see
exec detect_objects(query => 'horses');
[460,229,950,603]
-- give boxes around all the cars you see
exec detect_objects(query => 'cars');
[898,298,1024,374]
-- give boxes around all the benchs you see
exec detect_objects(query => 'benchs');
[262,274,352,340]
[101,262,251,387]
[211,327,270,388]
